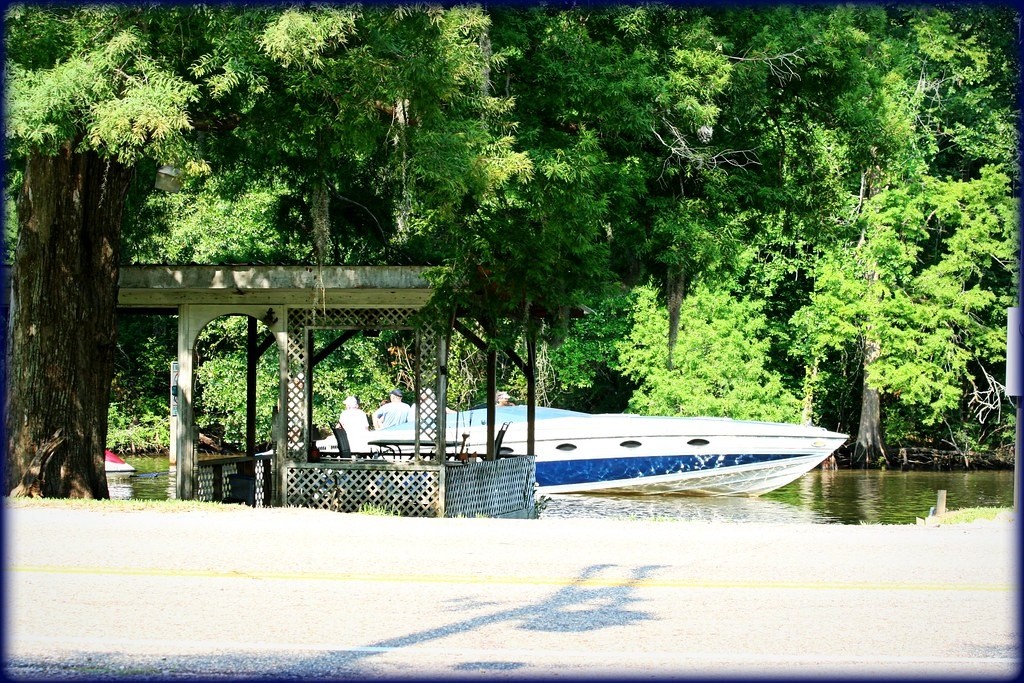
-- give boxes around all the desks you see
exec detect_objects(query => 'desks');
[367,440,462,463]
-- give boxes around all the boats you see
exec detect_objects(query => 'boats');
[254,404,850,499]
[105,449,138,472]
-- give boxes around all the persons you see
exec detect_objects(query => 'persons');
[336,396,369,433]
[496,391,515,406]
[372,387,457,434]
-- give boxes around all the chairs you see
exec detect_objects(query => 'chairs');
[328,420,363,461]
[469,421,513,463]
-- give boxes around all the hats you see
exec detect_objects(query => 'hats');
[390,389,404,397]
[342,396,358,405]
[501,394,511,400]
